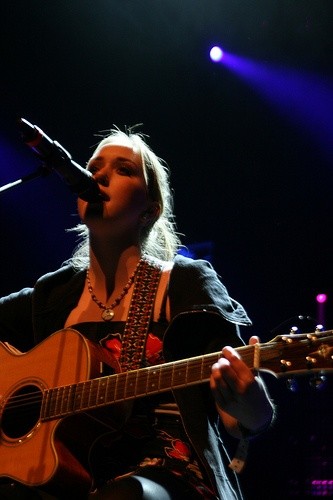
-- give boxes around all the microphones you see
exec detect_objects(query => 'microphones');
[17,117,101,204]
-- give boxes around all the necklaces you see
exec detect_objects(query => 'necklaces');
[86,257,145,321]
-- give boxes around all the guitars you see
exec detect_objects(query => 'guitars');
[0,323,332,487]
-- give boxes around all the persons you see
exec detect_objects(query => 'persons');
[0,122,280,500]
